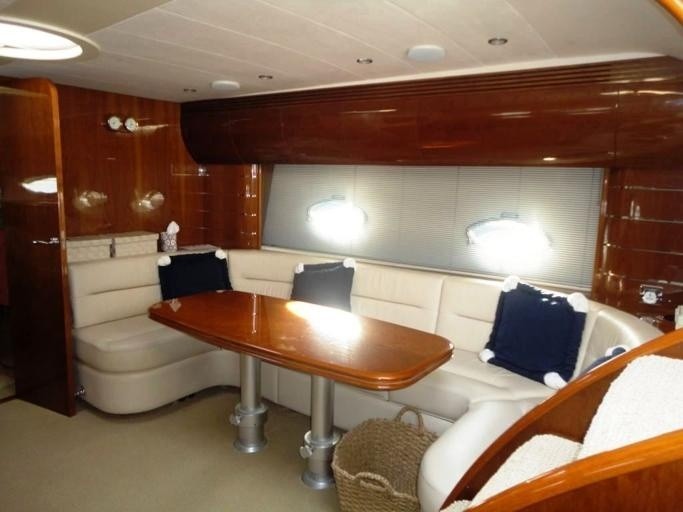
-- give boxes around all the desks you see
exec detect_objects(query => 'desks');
[145,287,455,490]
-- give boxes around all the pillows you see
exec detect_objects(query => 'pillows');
[156,249,234,301]
[288,257,355,312]
[475,271,591,394]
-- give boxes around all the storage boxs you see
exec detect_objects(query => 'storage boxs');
[100,229,159,258]
[64,234,112,264]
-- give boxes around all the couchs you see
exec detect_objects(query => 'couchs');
[64,246,668,512]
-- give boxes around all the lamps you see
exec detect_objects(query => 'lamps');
[465,211,553,268]
[306,194,368,243]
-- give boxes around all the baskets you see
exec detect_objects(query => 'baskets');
[329,402,439,511]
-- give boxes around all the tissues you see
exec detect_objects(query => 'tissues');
[160,221,180,252]
